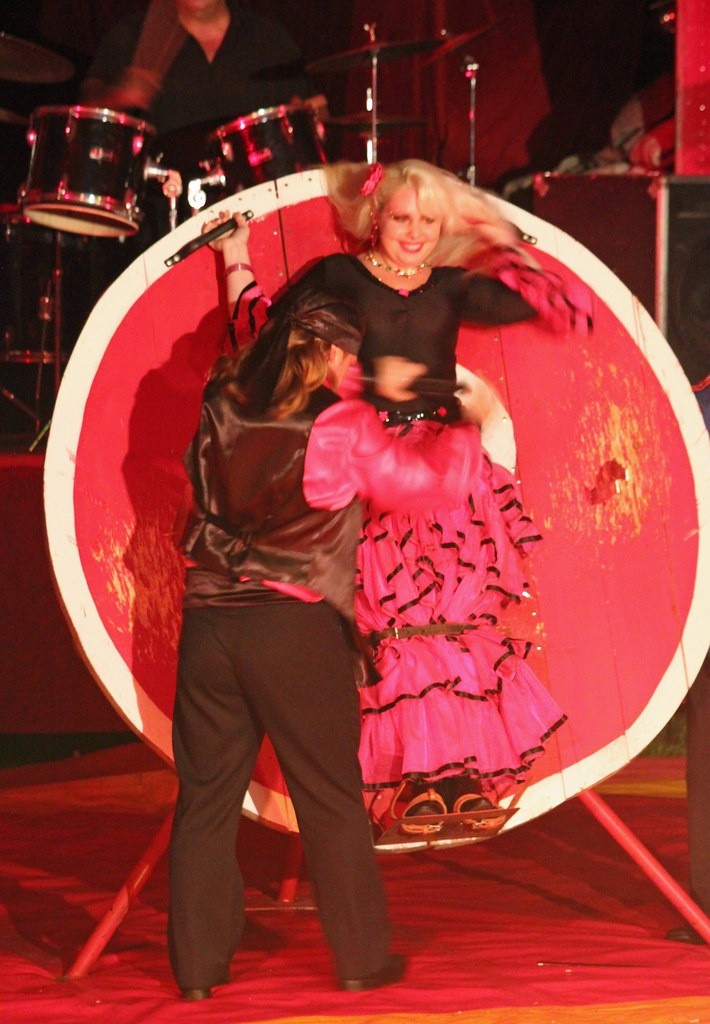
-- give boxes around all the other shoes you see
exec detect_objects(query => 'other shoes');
[415,783,439,825]
[440,777,492,810]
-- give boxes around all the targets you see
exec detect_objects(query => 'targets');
[38,160,710,853]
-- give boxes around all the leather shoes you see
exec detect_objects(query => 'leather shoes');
[339,954,404,988]
[183,988,209,1001]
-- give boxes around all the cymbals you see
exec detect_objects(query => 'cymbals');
[329,110,429,128]
[306,31,446,77]
[412,13,513,76]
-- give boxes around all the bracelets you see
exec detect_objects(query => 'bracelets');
[222,261,253,275]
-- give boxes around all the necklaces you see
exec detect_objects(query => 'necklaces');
[364,248,425,277]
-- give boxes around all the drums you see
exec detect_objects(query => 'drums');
[0,205,95,366]
[206,98,330,200]
[19,102,164,240]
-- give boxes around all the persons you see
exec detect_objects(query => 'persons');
[267,160,598,841]
[166,212,494,1005]
[108,0,332,224]
